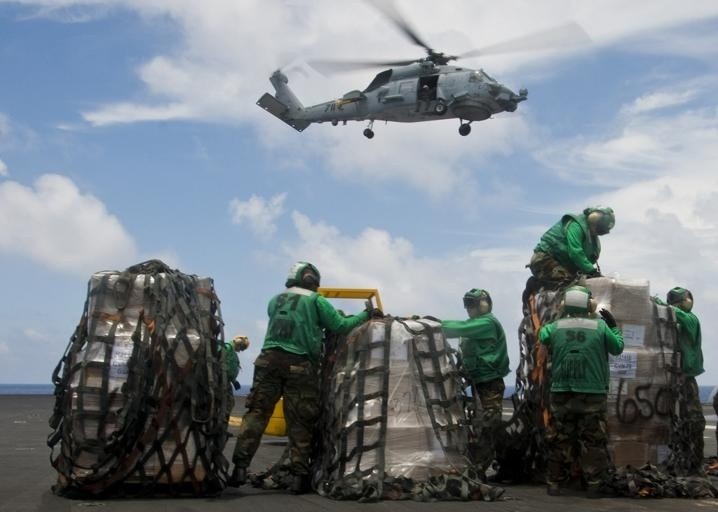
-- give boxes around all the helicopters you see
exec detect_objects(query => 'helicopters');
[254,1,590,140]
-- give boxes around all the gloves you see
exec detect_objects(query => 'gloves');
[365,307,383,318]
[599,308,616,327]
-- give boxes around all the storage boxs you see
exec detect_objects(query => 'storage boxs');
[51,266,699,501]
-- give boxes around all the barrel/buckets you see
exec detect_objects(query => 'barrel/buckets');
[263,399,288,437]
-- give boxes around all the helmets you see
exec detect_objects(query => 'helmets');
[564,286,595,314]
[285,261,320,290]
[464,289,492,318]
[584,207,615,235]
[234,336,249,350]
[667,287,694,311]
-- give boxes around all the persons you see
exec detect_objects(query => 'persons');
[533,284,628,500]
[221,334,249,421]
[649,283,707,458]
[418,285,511,484]
[517,202,618,304]
[222,258,386,497]
[411,83,435,113]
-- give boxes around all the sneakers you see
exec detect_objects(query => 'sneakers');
[587,485,614,498]
[292,475,309,493]
[546,480,559,495]
[230,468,245,487]
